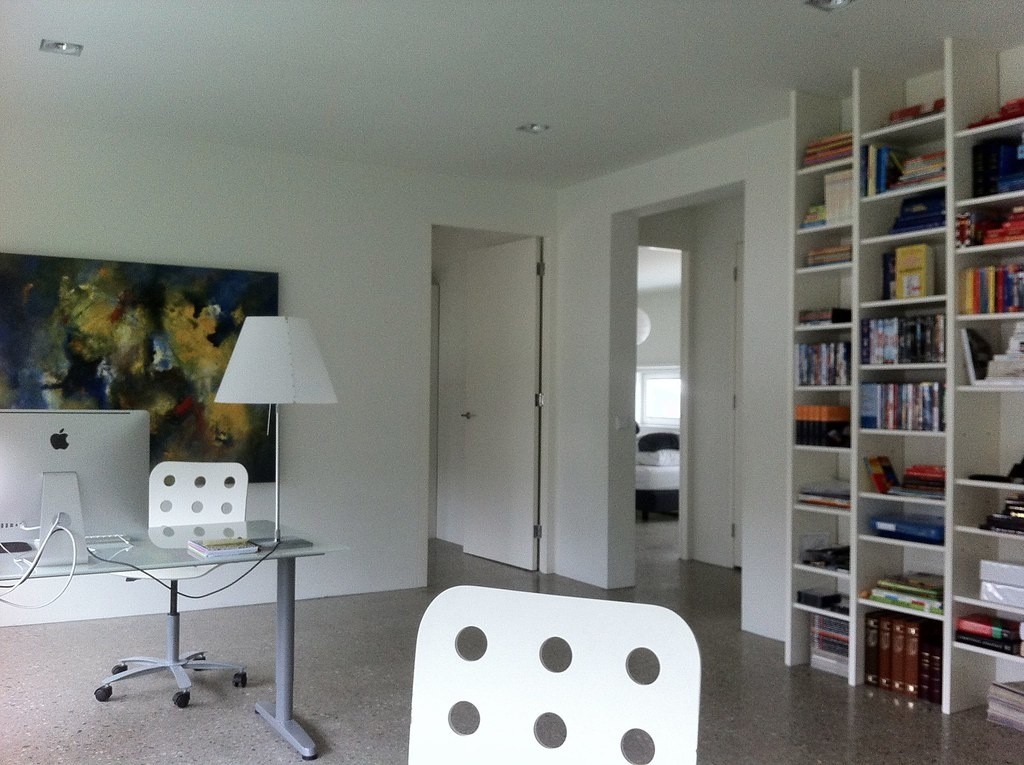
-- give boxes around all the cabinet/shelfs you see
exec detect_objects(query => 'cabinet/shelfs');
[785,36,1024,715]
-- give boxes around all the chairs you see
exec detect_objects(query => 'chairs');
[91,461,250,708]
[406,584,702,765]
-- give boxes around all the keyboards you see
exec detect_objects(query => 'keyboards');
[35,534,134,552]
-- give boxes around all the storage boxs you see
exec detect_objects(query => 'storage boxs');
[894,244,934,299]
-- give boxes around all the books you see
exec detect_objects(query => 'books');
[797,589,845,613]
[808,612,850,679]
[797,307,853,326]
[875,96,945,122]
[955,99,1024,730]
[861,313,945,364]
[187,537,259,558]
[794,342,850,386]
[860,571,944,616]
[859,381,942,431]
[860,612,943,704]
[798,493,850,510]
[856,139,946,191]
[795,404,850,447]
[801,130,856,164]
[804,243,852,265]
[795,167,856,227]
[869,243,935,298]
[862,456,945,498]
[867,514,945,544]
[885,190,946,232]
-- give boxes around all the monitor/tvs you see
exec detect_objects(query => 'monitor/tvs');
[0,409,151,568]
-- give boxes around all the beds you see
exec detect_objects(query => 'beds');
[634,465,680,525]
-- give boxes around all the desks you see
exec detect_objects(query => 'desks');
[0,518,352,761]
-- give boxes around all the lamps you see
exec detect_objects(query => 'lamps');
[636,308,652,344]
[212,316,339,552]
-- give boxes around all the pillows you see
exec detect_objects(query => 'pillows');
[638,449,679,466]
[638,432,679,452]
